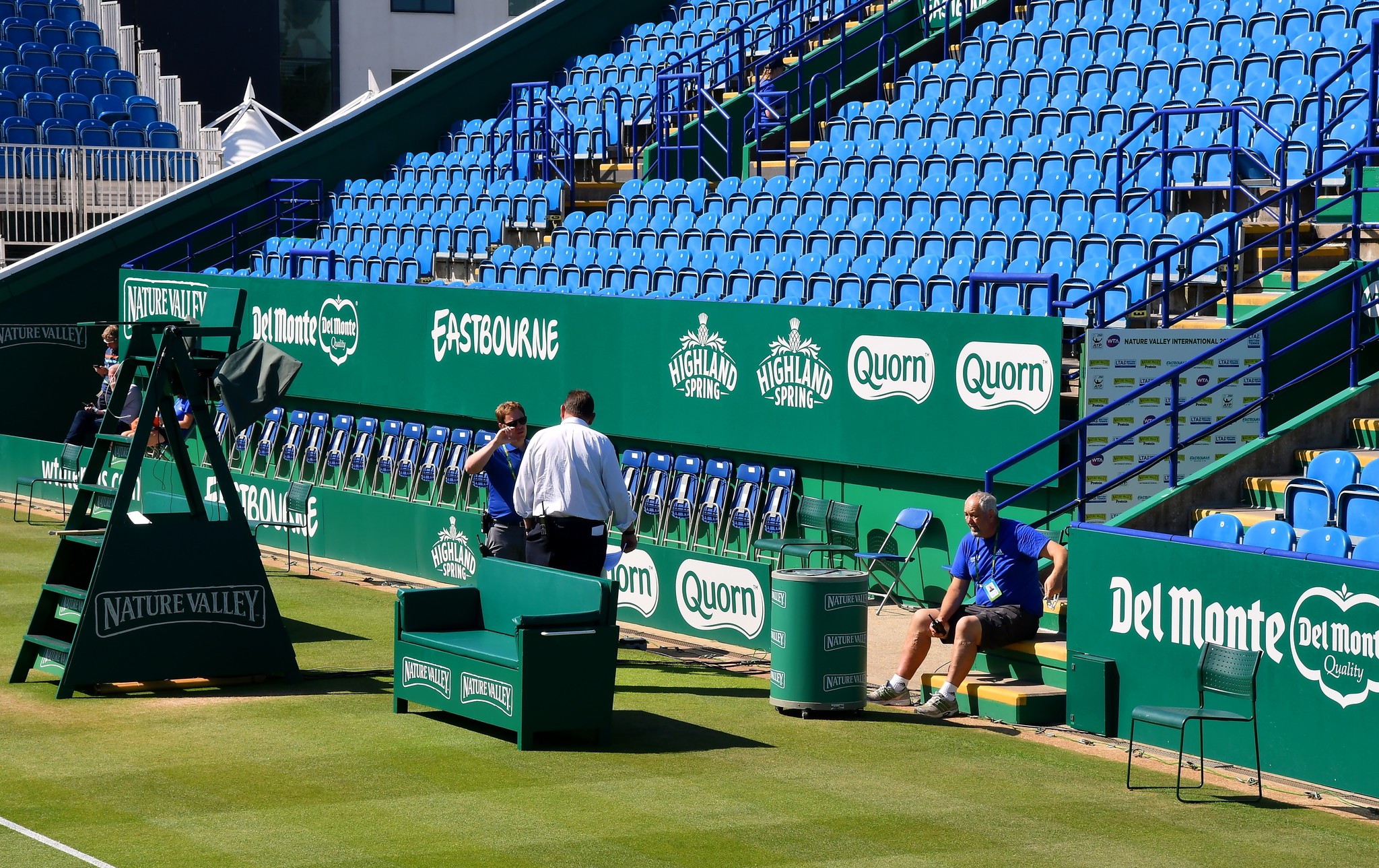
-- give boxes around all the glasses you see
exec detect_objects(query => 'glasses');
[500,415,527,427]
[103,337,118,344]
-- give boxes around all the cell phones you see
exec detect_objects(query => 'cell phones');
[621,542,627,553]
[82,402,99,412]
[93,365,100,369]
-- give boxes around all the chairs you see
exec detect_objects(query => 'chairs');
[435,427,476,509]
[130,285,248,379]
[754,496,862,571]
[204,0,1379,358]
[756,464,797,571]
[0,0,198,182]
[607,448,647,537]
[342,416,379,493]
[370,418,404,498]
[410,425,450,506]
[660,453,705,550]
[635,451,675,545]
[318,414,355,489]
[200,400,330,486]
[1127,641,1262,804]
[390,422,426,502]
[691,457,734,555]
[247,481,314,578]
[854,509,933,615]
[14,442,84,526]
[463,429,497,512]
[720,463,767,560]
[1194,451,1379,563]
[145,423,197,461]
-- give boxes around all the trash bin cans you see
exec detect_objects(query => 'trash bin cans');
[770,568,869,719]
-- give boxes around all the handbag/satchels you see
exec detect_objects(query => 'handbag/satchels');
[131,415,159,432]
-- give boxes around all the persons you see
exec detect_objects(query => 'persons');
[513,389,637,578]
[867,491,1068,719]
[63,364,143,446]
[465,401,530,563]
[121,392,194,447]
[93,325,119,411]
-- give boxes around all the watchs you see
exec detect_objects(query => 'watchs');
[622,528,635,536]
[525,526,535,532]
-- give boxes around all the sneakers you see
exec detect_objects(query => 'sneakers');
[867,680,911,706]
[914,691,959,718]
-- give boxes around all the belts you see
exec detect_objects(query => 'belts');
[491,520,525,527]
[537,517,589,524]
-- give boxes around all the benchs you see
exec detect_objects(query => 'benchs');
[393,556,620,751]
